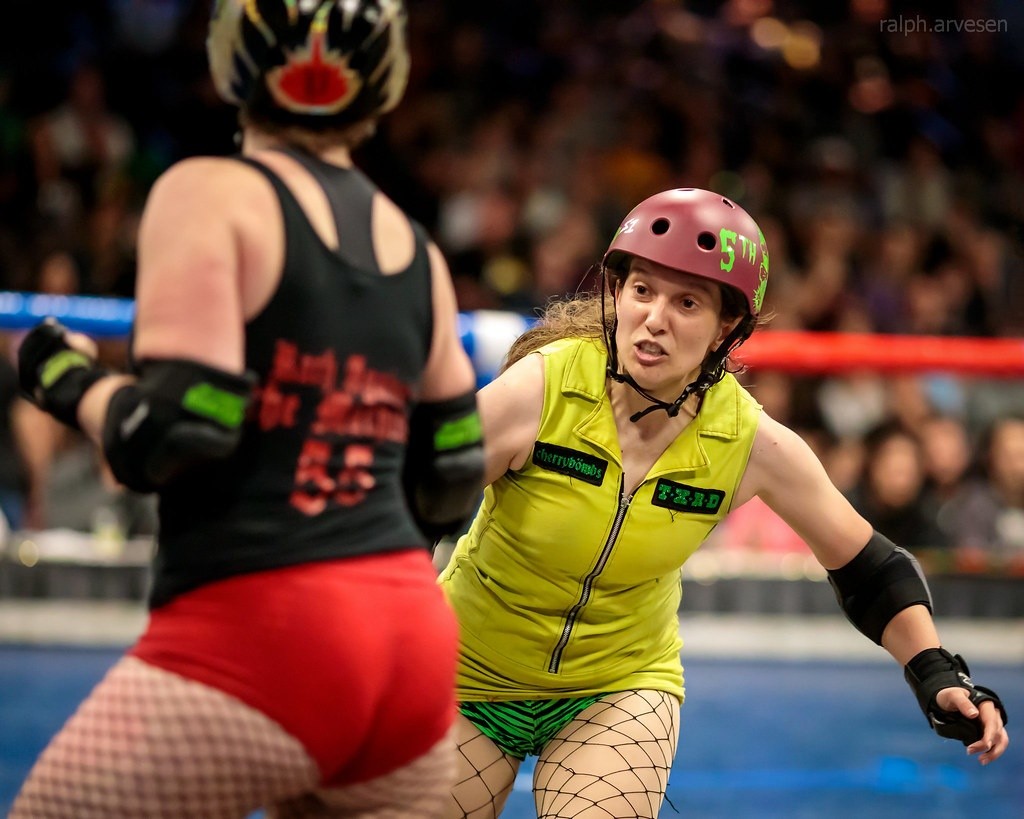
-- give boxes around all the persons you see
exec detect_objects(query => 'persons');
[435,187,1014,818]
[1,0,485,818]
[0,0,1024,564]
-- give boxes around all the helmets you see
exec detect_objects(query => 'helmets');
[600,188,770,318]
[206,0,411,145]
